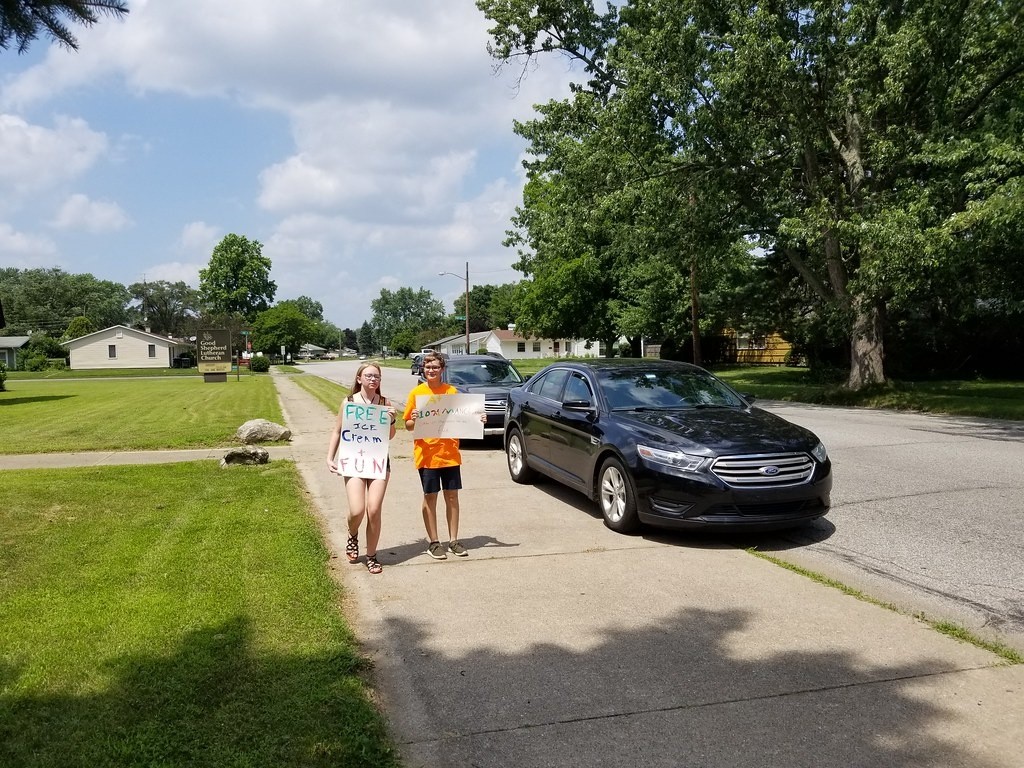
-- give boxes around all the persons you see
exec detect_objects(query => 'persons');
[402,351,487,559]
[326,363,398,574]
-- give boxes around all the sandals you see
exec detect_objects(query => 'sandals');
[345,530,359,561]
[365,554,382,574]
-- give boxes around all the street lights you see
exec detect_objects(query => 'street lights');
[439,272,469,354]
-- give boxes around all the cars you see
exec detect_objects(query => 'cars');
[411,355,428,375]
[320,355,335,360]
[504,361,833,535]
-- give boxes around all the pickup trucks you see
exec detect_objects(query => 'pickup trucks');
[409,349,447,361]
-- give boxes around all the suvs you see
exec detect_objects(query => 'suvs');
[416,352,531,441]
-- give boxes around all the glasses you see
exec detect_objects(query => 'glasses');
[363,374,380,381]
[425,365,440,372]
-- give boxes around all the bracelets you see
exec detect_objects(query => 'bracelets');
[391,419,396,425]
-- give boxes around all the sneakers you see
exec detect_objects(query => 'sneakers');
[448,540,468,556]
[427,541,447,559]
